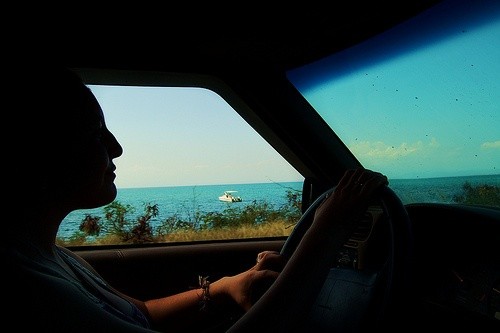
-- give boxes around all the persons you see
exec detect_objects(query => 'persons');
[0,65,387,333]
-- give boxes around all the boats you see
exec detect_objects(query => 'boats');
[218,191,242,203]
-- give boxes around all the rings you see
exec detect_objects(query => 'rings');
[256,257,262,263]
[354,181,364,187]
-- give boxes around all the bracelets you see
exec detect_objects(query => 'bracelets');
[195,275,210,309]
